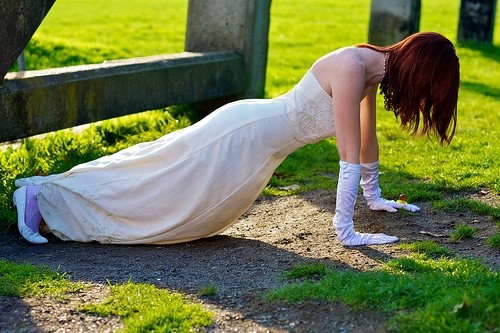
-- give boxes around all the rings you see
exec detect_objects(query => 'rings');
[396,192,408,204]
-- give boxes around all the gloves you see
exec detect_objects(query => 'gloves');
[360,161,420,212]
[333,160,399,246]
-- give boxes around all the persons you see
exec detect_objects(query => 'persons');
[15,31,463,247]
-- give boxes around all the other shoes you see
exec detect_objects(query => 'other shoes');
[12,186,48,243]
[15,175,39,187]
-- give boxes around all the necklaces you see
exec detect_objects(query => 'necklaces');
[384,52,391,111]
